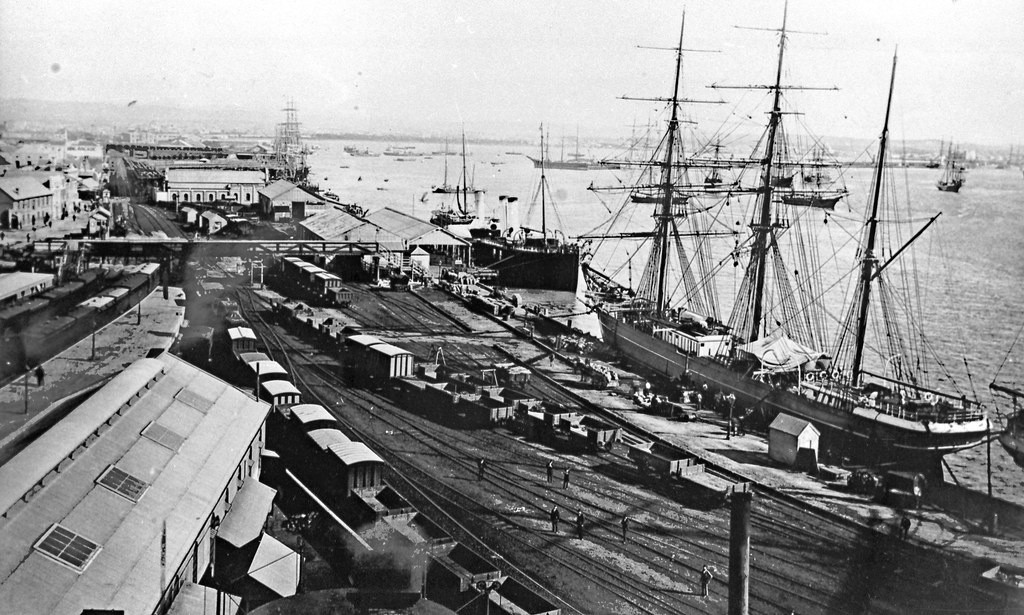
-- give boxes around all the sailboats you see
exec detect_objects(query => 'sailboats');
[582,1,1010,481]
[988,321,1024,470]
[526,121,619,170]
[936,142,963,192]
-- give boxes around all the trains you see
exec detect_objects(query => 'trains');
[1,230,167,370]
[276,300,624,452]
[217,294,564,615]
[278,256,350,309]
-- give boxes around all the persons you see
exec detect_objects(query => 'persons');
[563,467,570,490]
[700,565,713,597]
[575,509,584,540]
[621,515,629,544]
[550,505,559,533]
[545,460,554,483]
[477,457,486,481]
[898,513,911,540]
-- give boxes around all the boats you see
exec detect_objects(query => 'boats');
[465,123,580,291]
